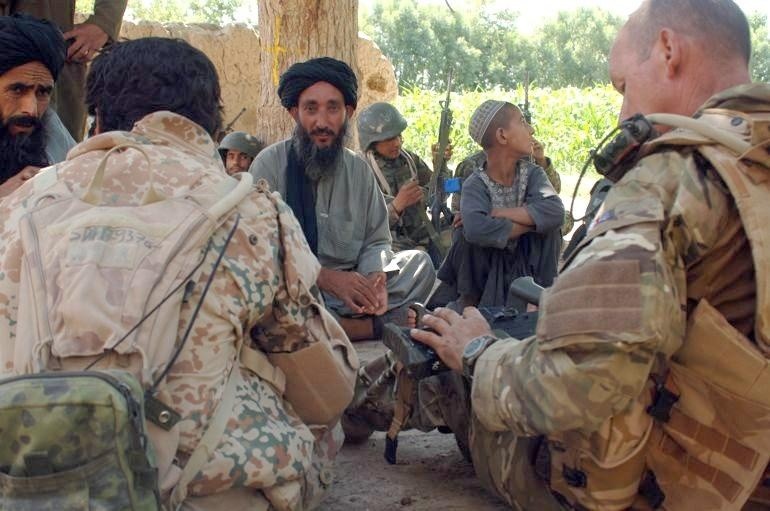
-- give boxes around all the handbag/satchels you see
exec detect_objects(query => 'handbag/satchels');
[0,368,159,511]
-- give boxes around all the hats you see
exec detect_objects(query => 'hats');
[468,99,506,144]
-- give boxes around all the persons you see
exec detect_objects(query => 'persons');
[215,131,263,176]
[0,13,77,205]
[0,1,128,143]
[247,56,437,342]
[449,135,562,213]
[426,100,564,316]
[1,37,359,510]
[341,1,769,510]
[355,101,452,270]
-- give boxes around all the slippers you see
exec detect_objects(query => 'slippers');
[372,302,427,340]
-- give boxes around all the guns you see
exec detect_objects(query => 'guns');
[217,108,246,145]
[515,64,532,125]
[382,276,550,381]
[429,69,457,233]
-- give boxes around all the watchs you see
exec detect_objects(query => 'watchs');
[460,334,501,389]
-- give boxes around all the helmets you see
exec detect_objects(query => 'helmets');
[357,103,407,152]
[219,132,259,157]
